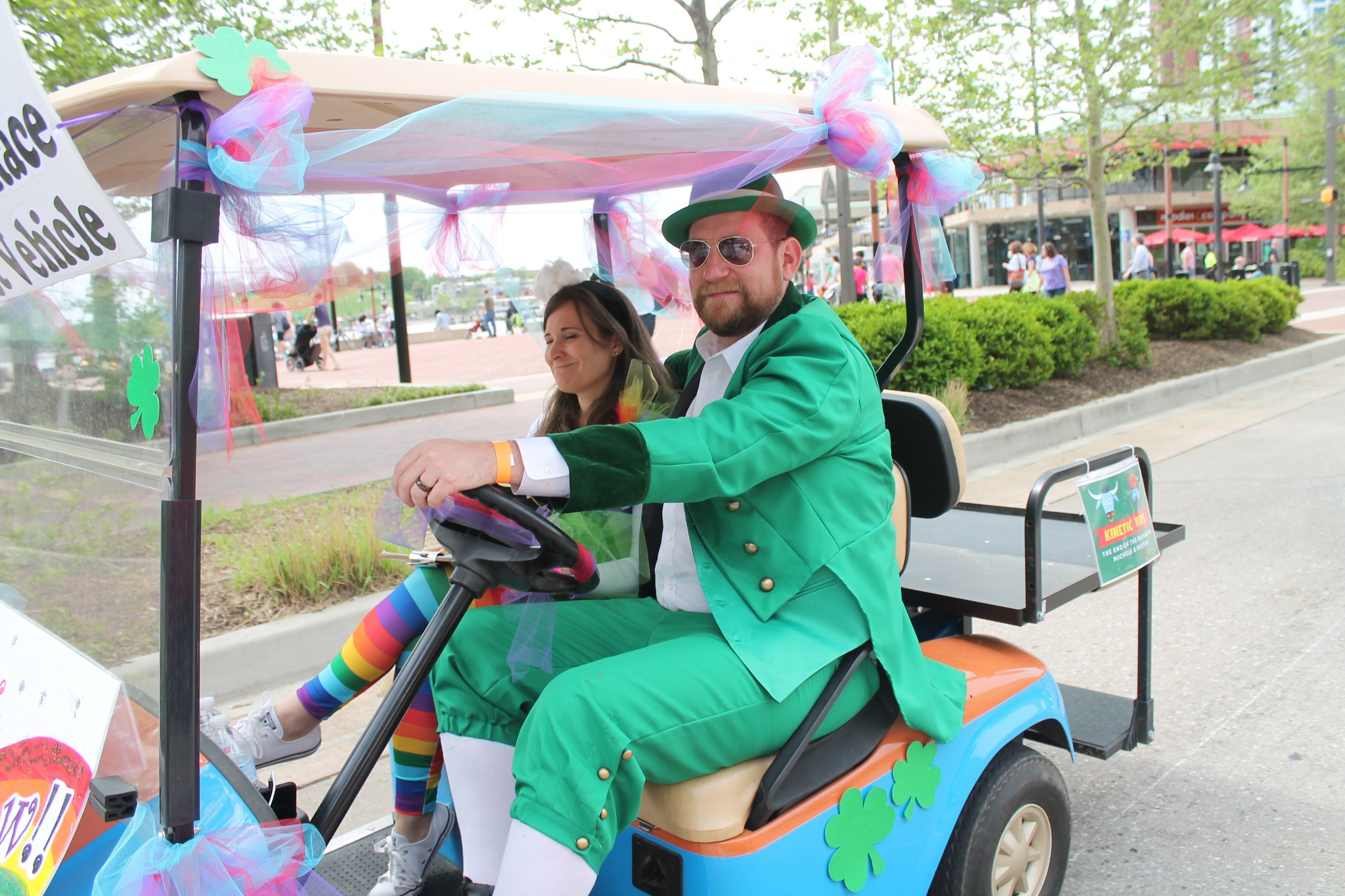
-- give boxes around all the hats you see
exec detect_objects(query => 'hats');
[661,164,817,251]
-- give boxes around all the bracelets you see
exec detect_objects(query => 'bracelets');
[490,433,513,485]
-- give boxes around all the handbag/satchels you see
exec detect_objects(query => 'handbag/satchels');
[1008,253,1025,289]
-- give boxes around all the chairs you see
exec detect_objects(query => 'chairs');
[633,454,914,843]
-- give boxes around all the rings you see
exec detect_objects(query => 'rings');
[416,477,432,492]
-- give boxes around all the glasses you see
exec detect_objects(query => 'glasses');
[679,236,788,270]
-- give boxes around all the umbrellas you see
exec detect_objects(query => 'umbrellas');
[1144,220,1326,263]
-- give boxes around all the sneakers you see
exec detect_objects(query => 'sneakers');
[236,704,323,769]
[367,802,456,896]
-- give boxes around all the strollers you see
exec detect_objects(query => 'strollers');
[466,317,490,340]
[285,322,326,373]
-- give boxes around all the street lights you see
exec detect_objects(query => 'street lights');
[1202,147,1224,283]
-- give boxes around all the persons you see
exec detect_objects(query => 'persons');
[1203,247,1217,282]
[504,300,525,335]
[219,276,676,896]
[1179,237,1195,278]
[306,289,340,372]
[269,299,297,361]
[389,160,966,896]
[481,288,498,338]
[1001,240,1071,297]
[433,308,452,332]
[824,249,870,305]
[355,314,374,349]
[807,274,820,296]
[379,300,395,330]
[1123,232,1157,283]
[1268,239,1282,279]
[1231,255,1265,280]
